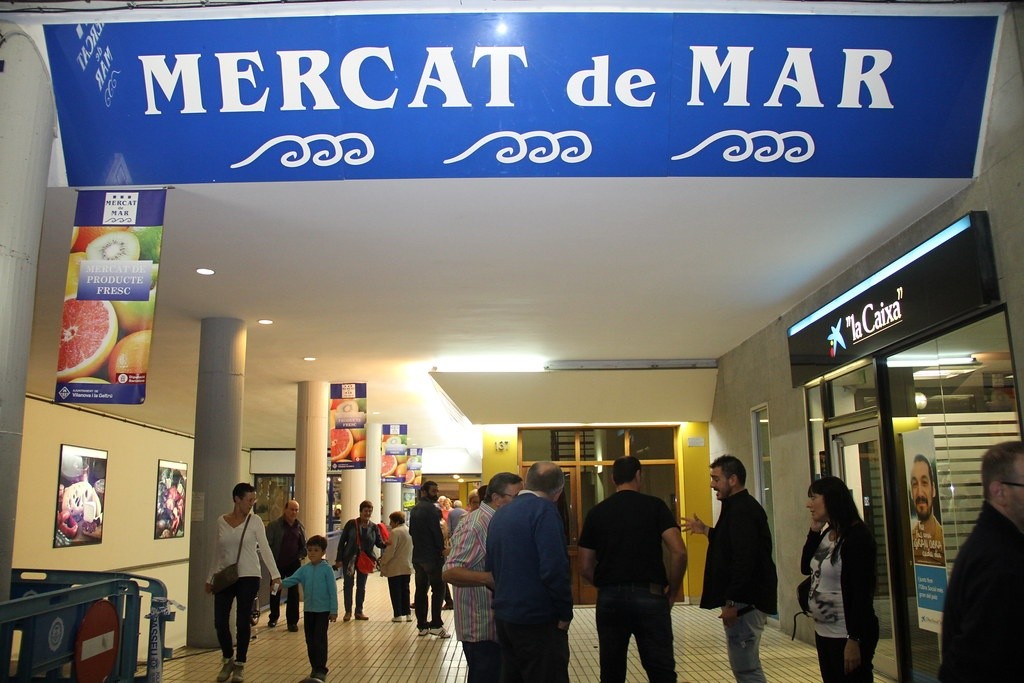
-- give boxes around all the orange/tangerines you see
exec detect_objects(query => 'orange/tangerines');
[57,226,164,384]
[381,434,407,477]
[329,399,367,463]
[403,456,421,485]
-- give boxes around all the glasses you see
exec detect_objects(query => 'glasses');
[504,493,518,498]
[243,497,256,502]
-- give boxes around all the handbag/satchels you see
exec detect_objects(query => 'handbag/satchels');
[357,551,374,574]
[796,576,812,616]
[212,564,238,594]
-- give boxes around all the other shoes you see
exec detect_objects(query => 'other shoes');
[430,627,451,638]
[392,615,402,622]
[419,628,429,635]
[287,624,298,632]
[311,671,326,683]
[234,661,244,682]
[217,659,233,682]
[268,620,277,627]
[406,615,412,621]
[343,612,351,620]
[442,603,453,609]
[410,603,415,608]
[355,613,368,620]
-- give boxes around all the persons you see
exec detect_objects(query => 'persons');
[335,509,341,519]
[408,480,450,638]
[409,508,453,610]
[441,472,522,683]
[448,500,468,535]
[460,488,480,521]
[440,497,454,522]
[205,482,282,683]
[265,500,307,632]
[486,461,573,683]
[335,501,390,621]
[908,454,945,566]
[435,495,446,509]
[679,455,778,683]
[579,456,688,682]
[270,535,338,683]
[938,442,1024,683]
[800,476,879,682]
[379,512,413,622]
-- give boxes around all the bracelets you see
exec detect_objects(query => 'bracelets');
[847,634,860,641]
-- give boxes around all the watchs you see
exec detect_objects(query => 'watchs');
[724,599,737,607]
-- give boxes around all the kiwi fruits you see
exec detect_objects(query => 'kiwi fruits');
[336,400,358,413]
[85,230,140,261]
[408,457,418,463]
[386,436,402,444]
